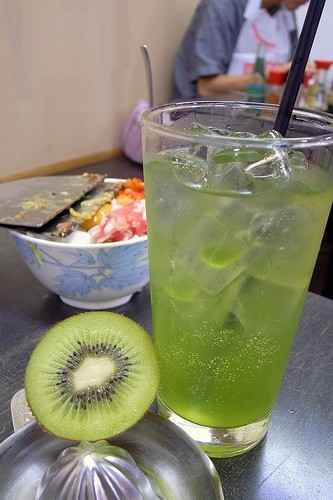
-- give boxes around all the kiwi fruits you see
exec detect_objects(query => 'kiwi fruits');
[25,311,158,442]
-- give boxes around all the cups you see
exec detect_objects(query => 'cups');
[141,99,333,457]
[121,102,174,164]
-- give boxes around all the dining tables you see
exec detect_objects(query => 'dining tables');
[0,154,333,500]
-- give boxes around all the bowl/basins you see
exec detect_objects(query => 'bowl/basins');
[7,176,150,309]
[0,409,225,500]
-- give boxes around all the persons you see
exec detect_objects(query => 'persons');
[172,0,315,111]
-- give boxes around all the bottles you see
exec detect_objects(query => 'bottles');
[246,43,333,113]
